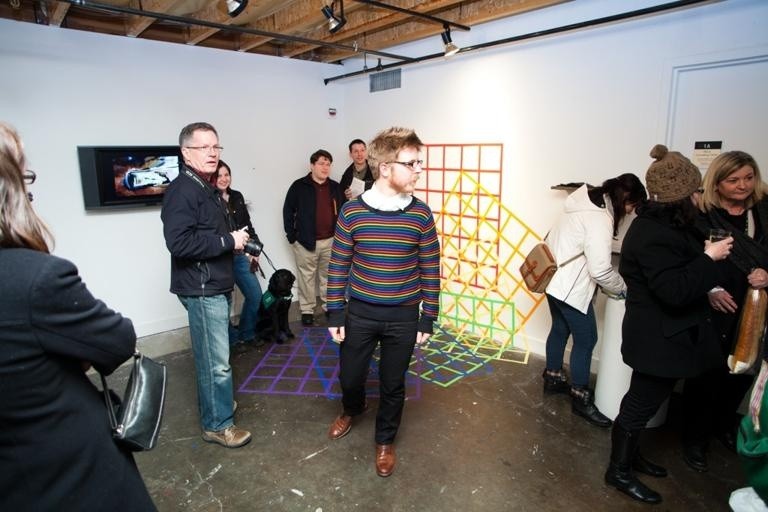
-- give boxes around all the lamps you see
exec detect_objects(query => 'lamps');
[318,0,348,34]
[439,23,460,58]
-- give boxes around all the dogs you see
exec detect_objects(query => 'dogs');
[255,269,296,345]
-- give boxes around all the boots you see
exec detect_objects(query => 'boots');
[570,387,612,428]
[632,441,667,478]
[543,369,572,396]
[605,419,661,504]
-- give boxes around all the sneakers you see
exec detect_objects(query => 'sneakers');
[244,337,264,347]
[228,343,247,353]
[202,425,252,448]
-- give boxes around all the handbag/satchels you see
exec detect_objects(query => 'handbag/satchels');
[113,352,167,452]
[519,244,557,293]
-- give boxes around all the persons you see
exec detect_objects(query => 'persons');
[684,148,768,476]
[325,126,444,479]
[0,117,159,510]
[603,142,734,504]
[210,159,267,352]
[339,135,380,209]
[283,148,341,327]
[162,121,253,448]
[541,170,648,428]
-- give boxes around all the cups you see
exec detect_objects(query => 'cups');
[709,229,732,243]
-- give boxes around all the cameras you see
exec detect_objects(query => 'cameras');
[243,238,264,257]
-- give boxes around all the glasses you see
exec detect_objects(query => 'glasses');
[22,170,36,185]
[385,160,423,171]
[186,144,224,152]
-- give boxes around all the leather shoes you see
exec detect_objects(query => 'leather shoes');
[302,314,313,324]
[329,400,368,440]
[376,444,397,477]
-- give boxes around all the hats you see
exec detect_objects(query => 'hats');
[646,144,701,203]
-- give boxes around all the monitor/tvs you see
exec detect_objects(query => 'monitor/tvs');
[76,144,184,210]
